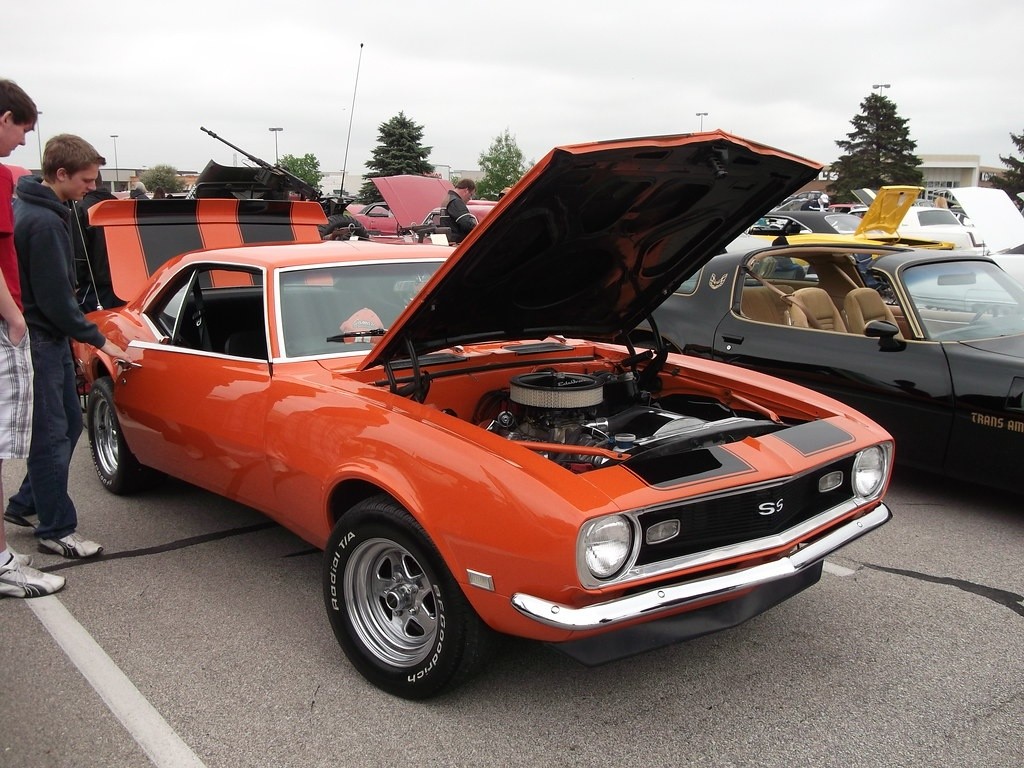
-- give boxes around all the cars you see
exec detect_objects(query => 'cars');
[111,158,499,244]
[74,129,896,704]
[634,235,1024,487]
[748,185,1024,303]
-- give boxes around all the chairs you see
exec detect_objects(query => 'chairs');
[842,288,907,342]
[224,329,263,358]
[784,287,848,333]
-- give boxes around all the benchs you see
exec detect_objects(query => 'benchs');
[172,285,358,353]
[741,285,795,325]
[749,229,787,235]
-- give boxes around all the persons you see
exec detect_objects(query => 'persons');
[70,171,174,314]
[933,197,948,209]
[809,194,830,211]
[4,134,133,559]
[0,78,67,601]
[439,179,479,244]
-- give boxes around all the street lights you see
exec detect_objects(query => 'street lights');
[697,112,708,132]
[873,84,890,96]
[270,128,283,164]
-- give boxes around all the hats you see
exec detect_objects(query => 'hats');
[821,194,829,203]
[134,181,145,191]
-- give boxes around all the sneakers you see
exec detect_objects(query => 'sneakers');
[0,552,34,577]
[0,566,66,598]
[3,505,40,529]
[38,531,104,559]
